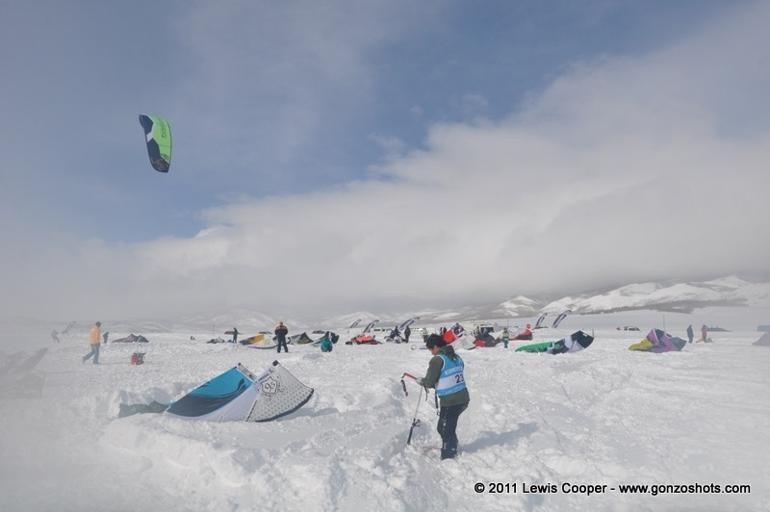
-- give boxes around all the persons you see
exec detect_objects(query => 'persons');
[233,327,237,343]
[403,325,411,344]
[417,333,471,459]
[275,321,289,354]
[394,325,399,335]
[81,321,103,365]
[686,324,694,344]
[702,324,710,341]
[501,327,509,347]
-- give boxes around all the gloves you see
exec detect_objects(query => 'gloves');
[415,377,424,386]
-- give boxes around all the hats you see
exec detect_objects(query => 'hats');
[424,333,447,349]
